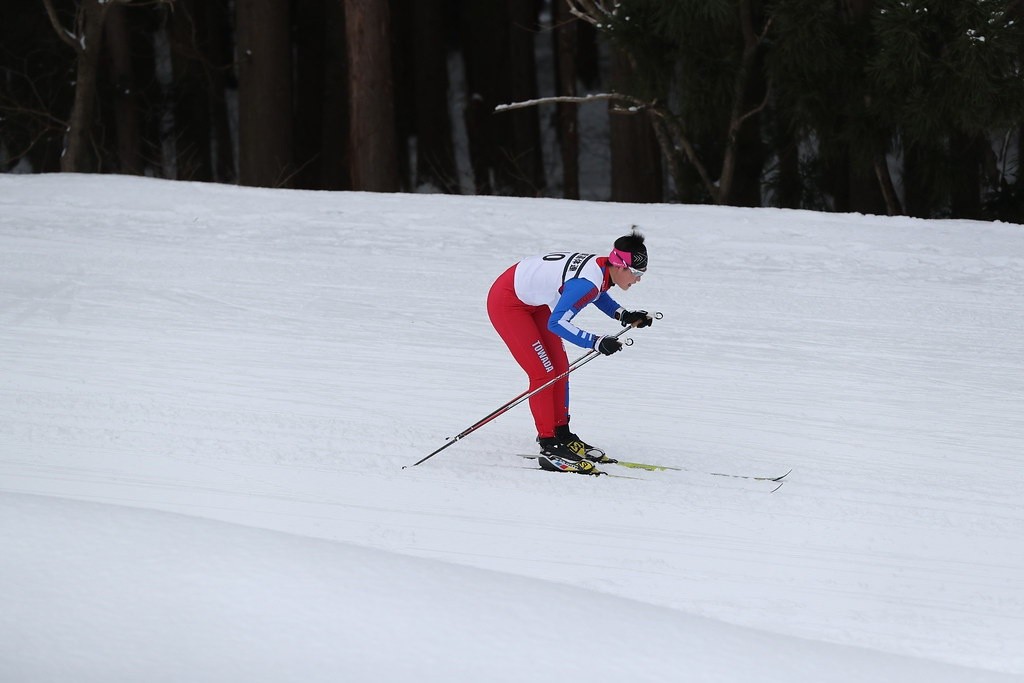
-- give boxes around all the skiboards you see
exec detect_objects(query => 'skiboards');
[514,452,793,494]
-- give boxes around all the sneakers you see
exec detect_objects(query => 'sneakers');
[538,444,596,474]
[556,433,605,462]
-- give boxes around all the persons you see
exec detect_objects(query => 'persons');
[487,232,653,473]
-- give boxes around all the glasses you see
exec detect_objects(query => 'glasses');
[628,267,644,278]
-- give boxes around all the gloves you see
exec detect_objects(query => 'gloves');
[592,334,622,356]
[620,310,653,328]
